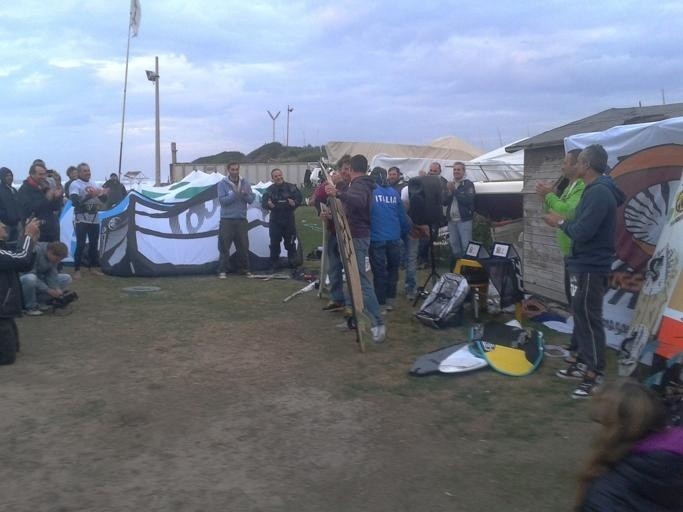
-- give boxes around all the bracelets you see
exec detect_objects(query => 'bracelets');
[556,218,565,228]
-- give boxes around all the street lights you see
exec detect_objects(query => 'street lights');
[144,55,165,191]
[286,104,297,147]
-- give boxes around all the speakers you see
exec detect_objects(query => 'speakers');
[407,175,443,225]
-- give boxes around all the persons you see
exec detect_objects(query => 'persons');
[572,377,681,512]
[311,150,475,343]
[215,160,256,281]
[534,147,587,362]
[259,168,307,278]
[539,142,628,400]
[0,154,124,365]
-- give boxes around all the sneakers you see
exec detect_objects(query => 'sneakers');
[38,303,53,310]
[219,272,227,279]
[244,272,254,279]
[272,270,279,275]
[556,357,588,380]
[292,270,302,277]
[323,286,417,343]
[571,367,605,399]
[24,309,42,315]
[92,270,104,277]
[74,271,81,280]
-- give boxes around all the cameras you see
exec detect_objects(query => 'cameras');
[27,218,47,228]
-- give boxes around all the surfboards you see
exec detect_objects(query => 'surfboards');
[619,170,683,378]
[318,160,365,353]
[411,320,544,379]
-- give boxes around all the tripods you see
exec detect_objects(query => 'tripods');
[412,225,442,308]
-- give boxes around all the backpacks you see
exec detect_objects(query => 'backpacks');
[416,273,470,328]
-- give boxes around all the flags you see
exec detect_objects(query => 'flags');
[128,0,141,38]
